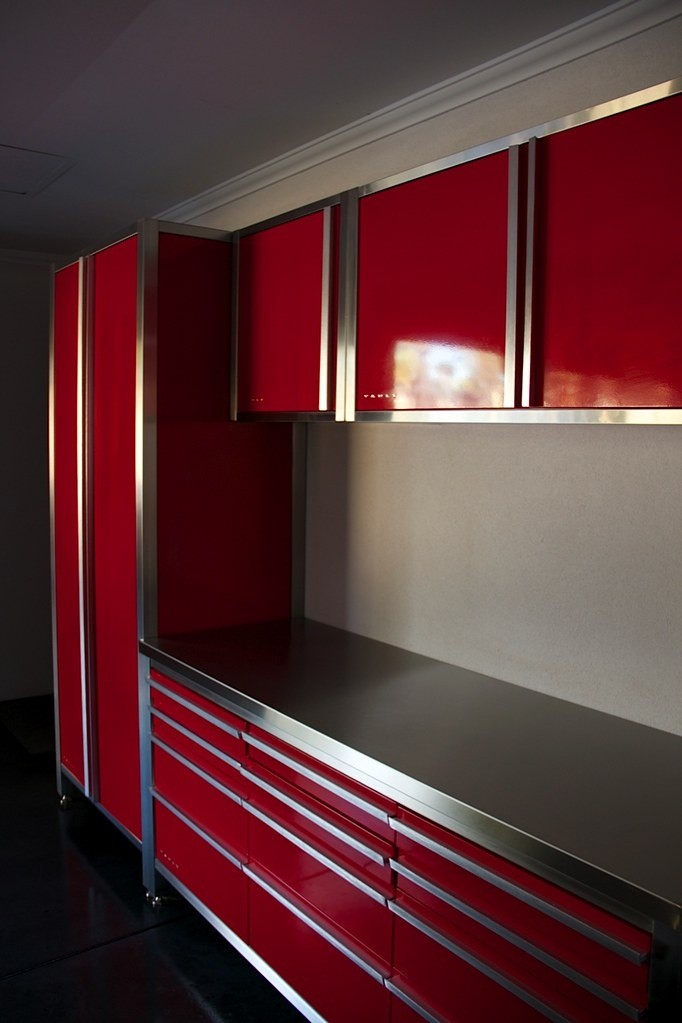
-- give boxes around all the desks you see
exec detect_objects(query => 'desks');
[137,616,682,931]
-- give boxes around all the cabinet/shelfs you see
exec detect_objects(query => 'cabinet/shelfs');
[229,191,345,423]
[343,75,682,426]
[147,666,249,959]
[237,723,398,1023]
[49,216,306,912]
[388,804,682,1023]
